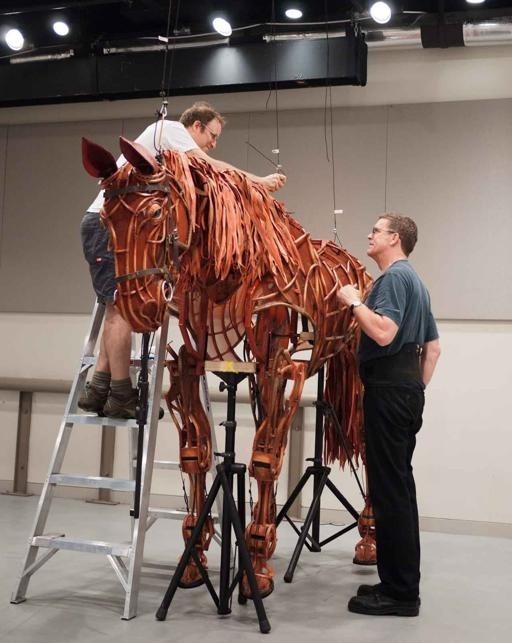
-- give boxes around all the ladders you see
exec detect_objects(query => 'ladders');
[10,293,235,621]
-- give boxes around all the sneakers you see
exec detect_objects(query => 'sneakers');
[99,386,164,422]
[76,380,106,414]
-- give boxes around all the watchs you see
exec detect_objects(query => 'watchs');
[350,300,363,316]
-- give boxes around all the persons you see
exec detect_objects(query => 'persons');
[336,212,442,617]
[80,101,287,421]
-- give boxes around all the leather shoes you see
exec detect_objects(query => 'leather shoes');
[347,582,421,617]
